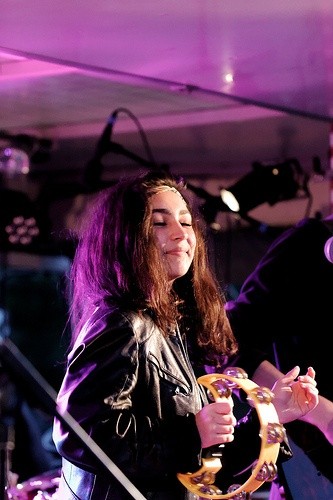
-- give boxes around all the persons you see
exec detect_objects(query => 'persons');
[204,214,331,499]
[48,173,320,500]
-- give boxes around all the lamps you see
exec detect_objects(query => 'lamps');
[221,165,285,215]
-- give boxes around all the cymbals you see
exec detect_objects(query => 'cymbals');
[173,365,289,500]
